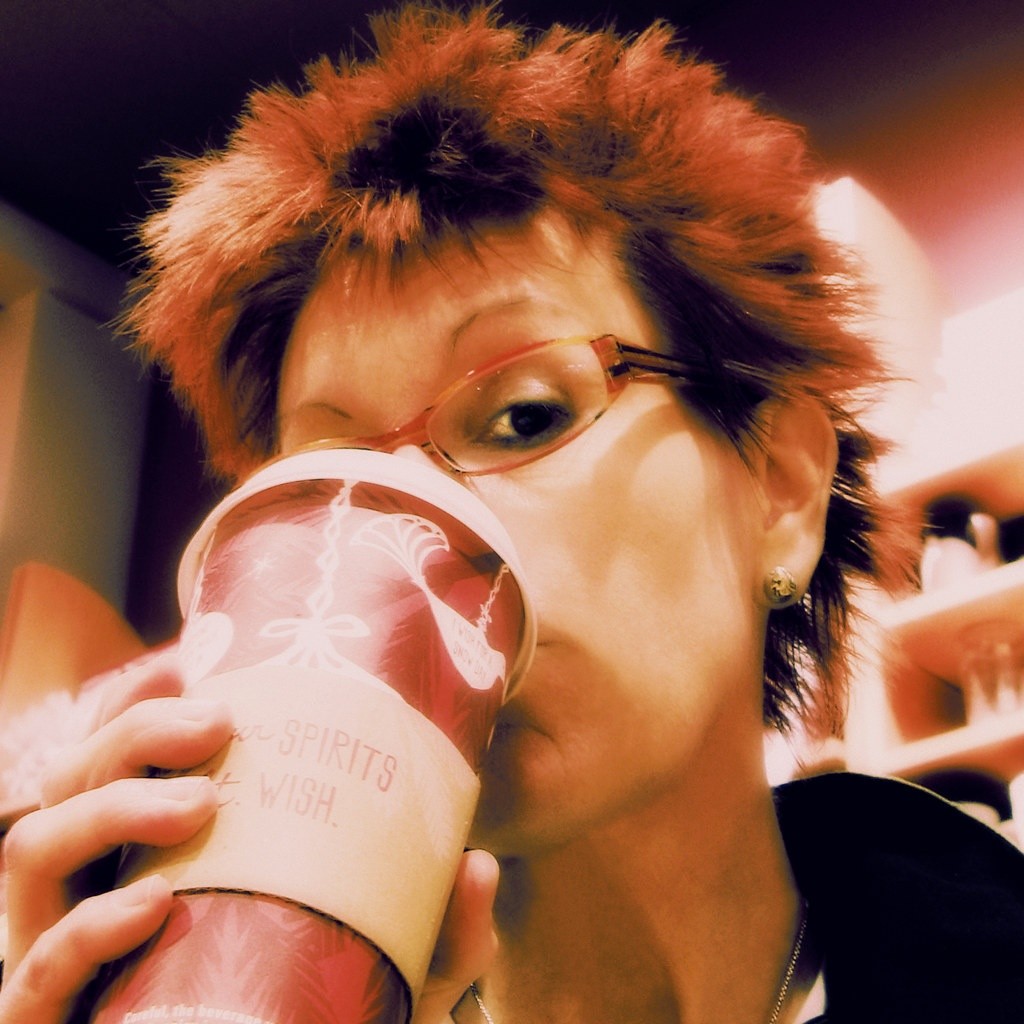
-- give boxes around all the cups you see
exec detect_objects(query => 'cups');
[83,444,537,1024]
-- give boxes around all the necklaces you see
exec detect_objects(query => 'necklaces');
[472,924,804,1023]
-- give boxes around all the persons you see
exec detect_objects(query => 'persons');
[0,0,1024,1022]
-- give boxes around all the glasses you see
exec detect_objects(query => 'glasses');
[246,336,711,476]
